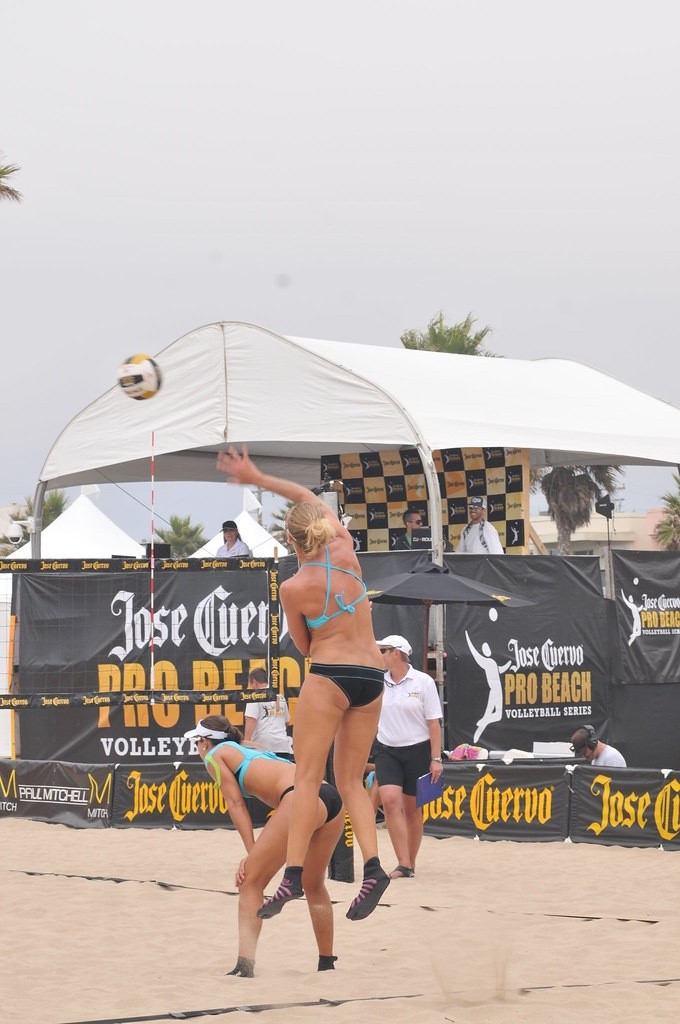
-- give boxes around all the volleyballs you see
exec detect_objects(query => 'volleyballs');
[117,353,162,401]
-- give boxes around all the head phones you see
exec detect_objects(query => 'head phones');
[581,724,599,750]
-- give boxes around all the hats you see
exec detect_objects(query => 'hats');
[375,635,412,657]
[572,728,591,757]
[219,521,236,532]
[467,496,483,508]
[184,719,229,739]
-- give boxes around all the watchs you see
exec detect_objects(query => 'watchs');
[431,757,442,763]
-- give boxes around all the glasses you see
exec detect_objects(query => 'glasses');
[469,505,484,511]
[408,520,421,525]
[380,647,394,654]
[195,734,212,746]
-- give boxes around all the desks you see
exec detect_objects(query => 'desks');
[0,750,679,852]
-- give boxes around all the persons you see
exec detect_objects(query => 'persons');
[217,445,391,920]
[570,725,628,767]
[376,635,442,880]
[395,510,454,552]
[454,497,505,554]
[363,763,381,817]
[217,520,250,557]
[185,668,346,978]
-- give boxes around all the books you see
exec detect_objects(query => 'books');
[416,769,443,808]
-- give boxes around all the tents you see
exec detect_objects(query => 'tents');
[0,494,146,691]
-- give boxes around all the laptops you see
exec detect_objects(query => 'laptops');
[411,528,431,550]
